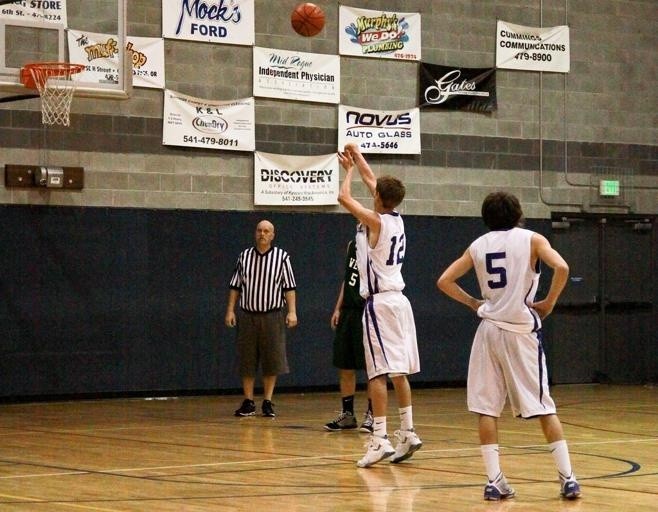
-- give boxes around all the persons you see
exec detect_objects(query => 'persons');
[334,142,426,469]
[223,219,299,419]
[433,189,584,502]
[322,213,376,435]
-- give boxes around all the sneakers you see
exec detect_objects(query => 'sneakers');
[357,436,396,467]
[233,400,256,416]
[261,404,275,417]
[390,430,422,463]
[484,472,515,500]
[324,414,357,431]
[359,413,374,433]
[559,471,580,497]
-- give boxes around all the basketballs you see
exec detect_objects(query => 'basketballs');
[290,2,326,36]
